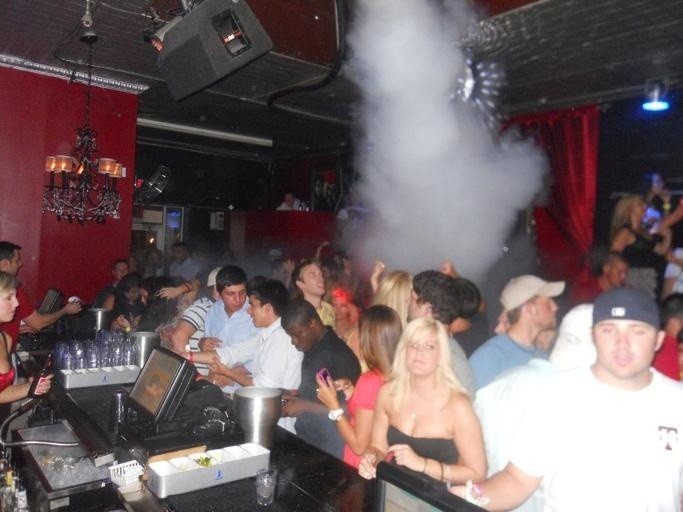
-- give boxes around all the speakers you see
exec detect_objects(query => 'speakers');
[157,0,275,102]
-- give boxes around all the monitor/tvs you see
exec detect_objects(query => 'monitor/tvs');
[125,345,198,432]
[38,288,65,315]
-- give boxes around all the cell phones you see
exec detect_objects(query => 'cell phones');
[318,367,335,387]
[280,397,288,408]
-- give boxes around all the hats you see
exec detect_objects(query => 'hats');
[499,272,567,312]
[206,267,224,288]
[592,286,662,333]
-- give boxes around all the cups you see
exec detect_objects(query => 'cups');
[52,329,134,373]
[256,469,278,506]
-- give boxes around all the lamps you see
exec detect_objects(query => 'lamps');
[41,29,127,225]
[639,72,672,111]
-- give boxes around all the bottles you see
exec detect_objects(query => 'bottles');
[28,354,53,399]
[0,454,32,512]
[112,390,128,424]
